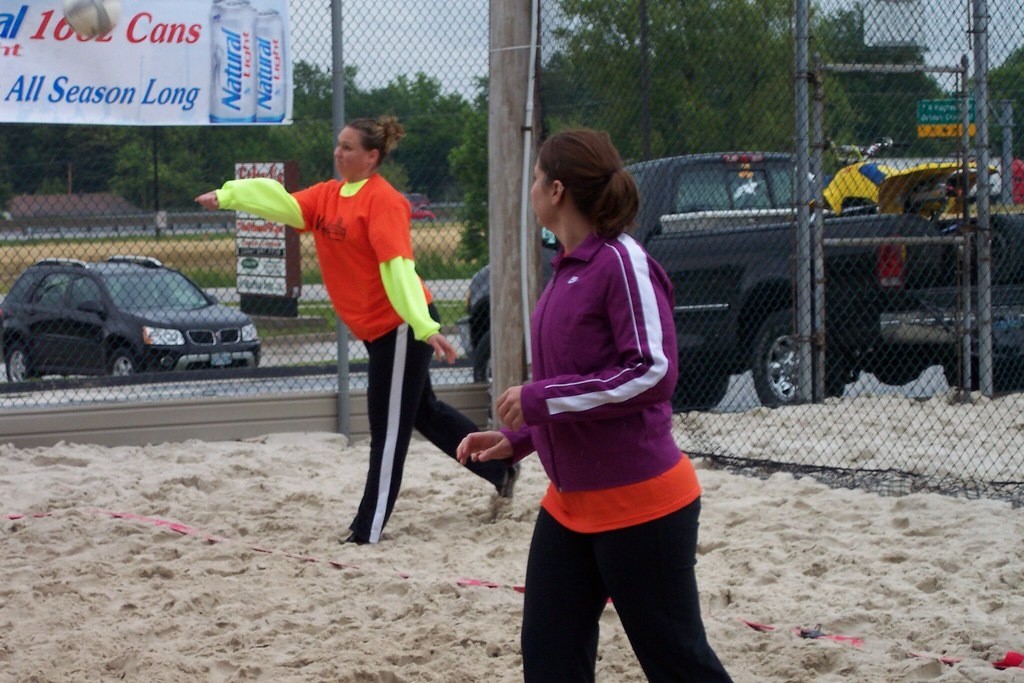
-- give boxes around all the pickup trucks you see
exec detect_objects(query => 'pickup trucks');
[462,147,1022,408]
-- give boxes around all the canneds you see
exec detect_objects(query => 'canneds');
[255,10,286,122]
[210,0,255,123]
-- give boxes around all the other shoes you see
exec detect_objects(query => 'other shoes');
[337,530,355,545]
[500,462,521,498]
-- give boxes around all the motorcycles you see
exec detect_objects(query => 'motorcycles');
[806,137,996,217]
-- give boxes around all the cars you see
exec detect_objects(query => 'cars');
[402,191,435,222]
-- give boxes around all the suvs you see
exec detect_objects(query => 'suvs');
[0,253,263,381]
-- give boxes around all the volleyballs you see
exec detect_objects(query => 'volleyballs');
[62,0,121,36]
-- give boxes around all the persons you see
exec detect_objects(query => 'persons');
[455,127,736,682]
[194,113,523,546]
